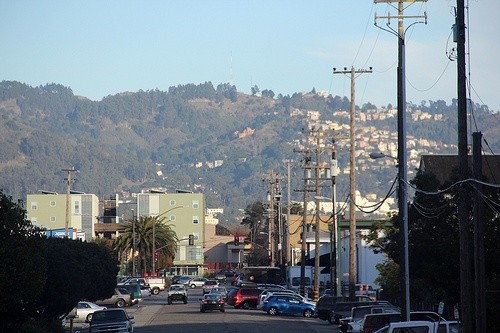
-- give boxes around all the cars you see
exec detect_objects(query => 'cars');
[85,308,136,333]
[188,277,210,289]
[91,276,166,308]
[215,273,227,284]
[170,275,192,286]
[209,287,228,303]
[198,293,226,313]
[65,301,108,324]
[226,266,462,333]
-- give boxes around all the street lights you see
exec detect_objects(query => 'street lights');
[260,231,275,267]
[238,208,266,266]
[314,195,338,296]
[368,152,412,333]
[130,208,135,277]
[152,205,185,278]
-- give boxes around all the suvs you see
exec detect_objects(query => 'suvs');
[166,284,188,305]
[202,280,221,296]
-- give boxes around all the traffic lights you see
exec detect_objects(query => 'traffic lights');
[234,236,240,246]
[154,252,158,262]
[155,269,160,273]
